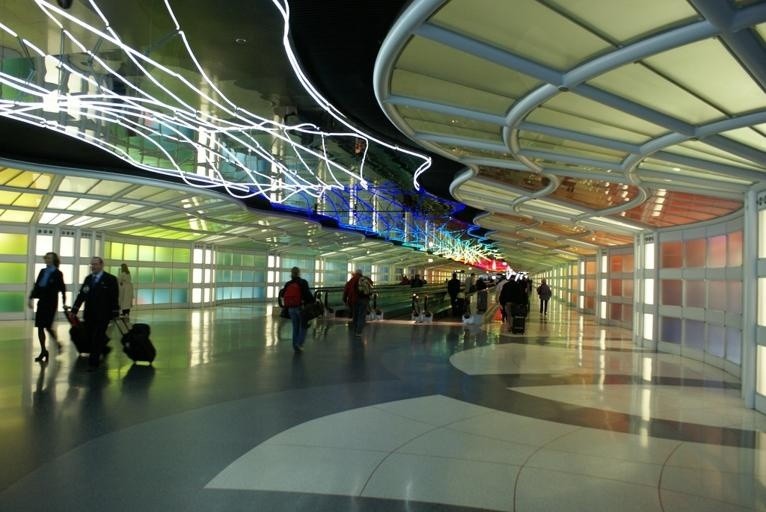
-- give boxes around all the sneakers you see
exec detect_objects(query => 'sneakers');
[295,341,304,354]
[354,332,363,338]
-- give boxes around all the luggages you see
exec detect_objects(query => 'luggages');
[64,306,92,354]
[512,316,525,335]
[452,297,465,317]
[115,316,156,364]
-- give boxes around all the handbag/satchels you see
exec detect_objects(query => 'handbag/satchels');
[510,302,526,316]
[301,298,324,321]
[457,292,465,299]
[358,275,375,298]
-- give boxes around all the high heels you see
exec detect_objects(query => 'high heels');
[35,350,48,362]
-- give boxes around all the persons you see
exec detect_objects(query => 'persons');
[68,256,120,367]
[343,268,374,338]
[25,251,66,362]
[398,272,428,311]
[117,263,134,316]
[445,272,552,333]
[282,266,316,353]
